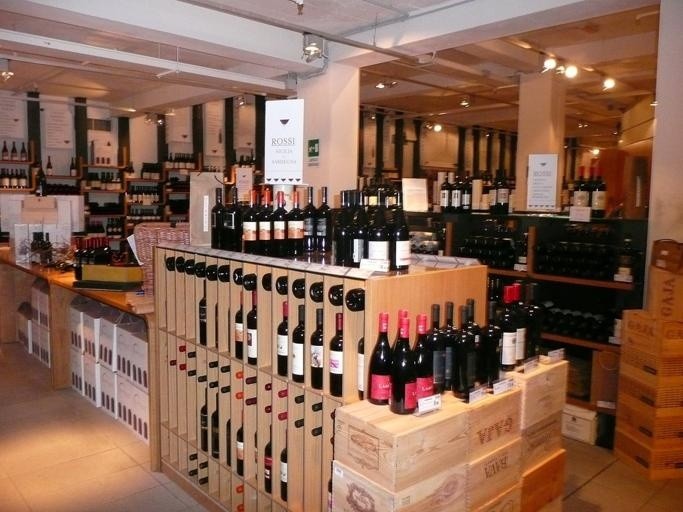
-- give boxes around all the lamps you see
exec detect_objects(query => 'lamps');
[0,57,16,80]
[233,90,251,113]
[304,33,323,60]
[509,33,618,95]
[460,95,470,106]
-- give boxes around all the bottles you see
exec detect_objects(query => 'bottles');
[569,163,607,217]
[43,153,124,235]
[162,330,331,512]
[366,276,541,415]
[29,232,54,267]
[238,155,256,169]
[200,164,221,173]
[437,170,508,214]
[125,152,196,222]
[209,175,411,271]
[162,252,346,401]
[0,138,28,189]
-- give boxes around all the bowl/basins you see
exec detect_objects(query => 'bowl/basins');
[72,237,112,280]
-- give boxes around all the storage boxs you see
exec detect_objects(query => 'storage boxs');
[613,240,683,481]
